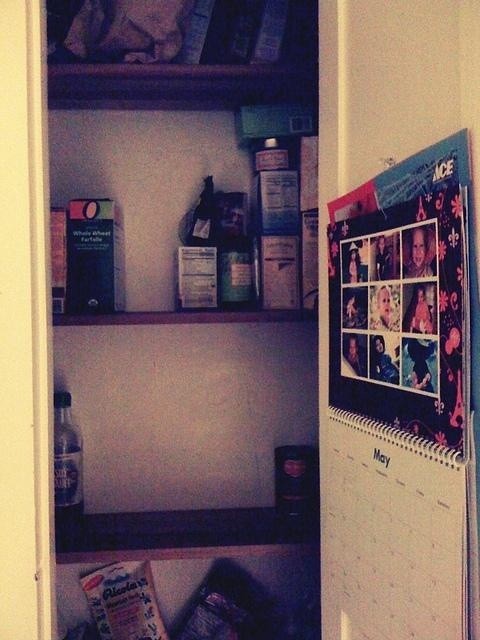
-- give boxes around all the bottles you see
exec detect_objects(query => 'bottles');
[53,390,84,528]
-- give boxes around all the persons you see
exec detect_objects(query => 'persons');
[341,220,436,392]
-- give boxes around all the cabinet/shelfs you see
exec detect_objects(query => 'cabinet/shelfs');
[47,50,328,565]
